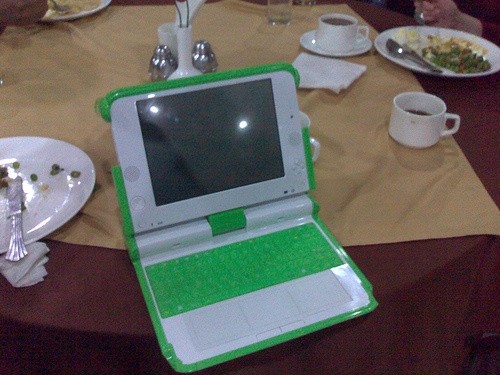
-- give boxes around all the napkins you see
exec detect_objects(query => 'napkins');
[289,51,368,93]
[0,242,52,288]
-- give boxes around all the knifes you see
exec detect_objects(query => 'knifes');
[6,177,27,262]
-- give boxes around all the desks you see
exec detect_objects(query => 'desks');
[0,0,500,375]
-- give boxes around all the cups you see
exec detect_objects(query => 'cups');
[316,13,369,53]
[157,23,178,60]
[298,110,321,164]
[388,92,461,149]
[267,0,293,26]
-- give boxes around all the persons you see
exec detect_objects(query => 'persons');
[414,0,500,47]
[0,0,48,36]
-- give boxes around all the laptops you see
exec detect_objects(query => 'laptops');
[98,62,379,370]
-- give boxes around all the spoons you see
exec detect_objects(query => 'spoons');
[386,38,437,71]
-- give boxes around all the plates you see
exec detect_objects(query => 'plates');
[0,135,96,255]
[299,29,372,58]
[39,0,112,24]
[373,25,500,77]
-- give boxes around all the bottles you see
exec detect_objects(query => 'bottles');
[149,46,178,80]
[191,41,218,73]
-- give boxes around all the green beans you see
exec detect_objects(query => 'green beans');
[421,45,491,74]
[13,161,80,180]
